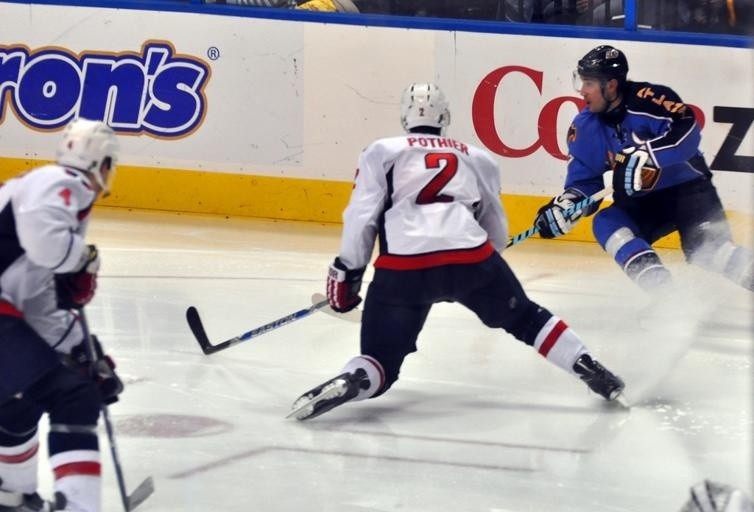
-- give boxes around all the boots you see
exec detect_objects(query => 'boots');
[573,355,623,399]
[0,491,52,512]
[292,373,357,421]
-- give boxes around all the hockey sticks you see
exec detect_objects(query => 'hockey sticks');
[186,298,331,353]
[78,308,156,512]
[311,187,614,324]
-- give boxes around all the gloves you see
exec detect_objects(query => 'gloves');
[327,258,362,313]
[612,145,647,198]
[68,243,99,306]
[535,192,574,239]
[72,337,123,400]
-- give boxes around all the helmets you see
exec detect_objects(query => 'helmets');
[56,118,120,170]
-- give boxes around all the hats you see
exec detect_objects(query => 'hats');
[577,45,628,78]
[400,81,450,129]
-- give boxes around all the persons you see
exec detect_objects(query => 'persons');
[291,81,625,422]
[0,120,119,512]
[535,46,753,294]
